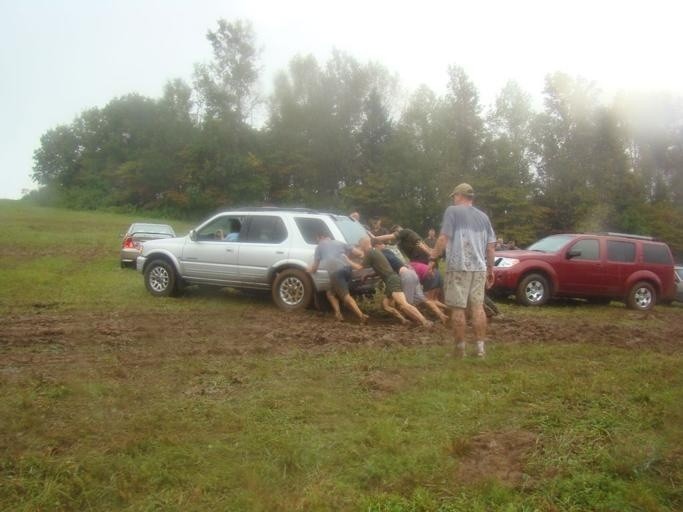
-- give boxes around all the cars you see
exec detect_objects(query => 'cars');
[135,206,384,310]
[119,223,175,268]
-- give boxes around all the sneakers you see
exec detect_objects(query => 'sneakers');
[359,314,370,326]
[336,314,343,322]
[444,349,485,357]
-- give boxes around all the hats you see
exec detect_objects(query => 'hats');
[448,183,474,198]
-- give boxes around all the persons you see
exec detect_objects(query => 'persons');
[304,230,367,324]
[216,218,240,242]
[505,240,520,250]
[340,210,450,330]
[425,182,497,360]
[496,238,505,249]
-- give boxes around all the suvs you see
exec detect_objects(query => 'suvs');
[484,232,683,310]
